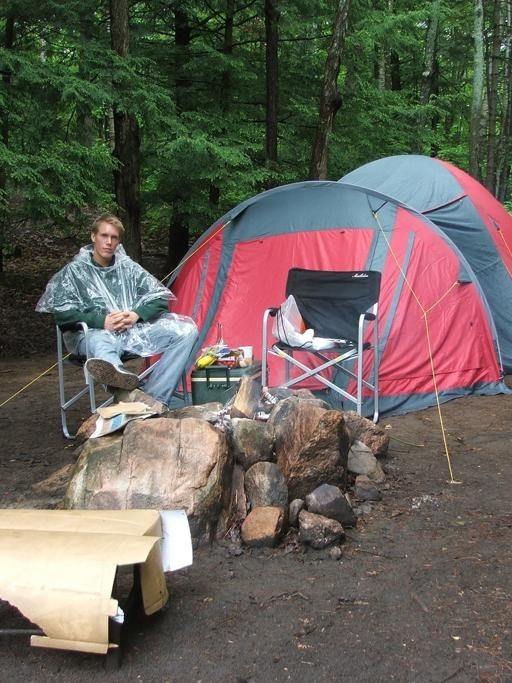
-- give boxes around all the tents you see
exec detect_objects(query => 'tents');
[338,155,512,374]
[141,180,512,422]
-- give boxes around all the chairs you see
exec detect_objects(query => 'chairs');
[55,312,189,439]
[255,268,382,424]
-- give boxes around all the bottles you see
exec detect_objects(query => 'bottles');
[215,322,224,346]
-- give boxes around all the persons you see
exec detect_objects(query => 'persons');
[35,212,199,408]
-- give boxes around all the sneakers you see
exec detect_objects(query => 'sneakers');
[86,358,140,391]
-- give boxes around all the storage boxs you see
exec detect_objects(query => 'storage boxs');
[190,360,269,407]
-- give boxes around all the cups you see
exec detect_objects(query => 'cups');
[238,347,253,367]
